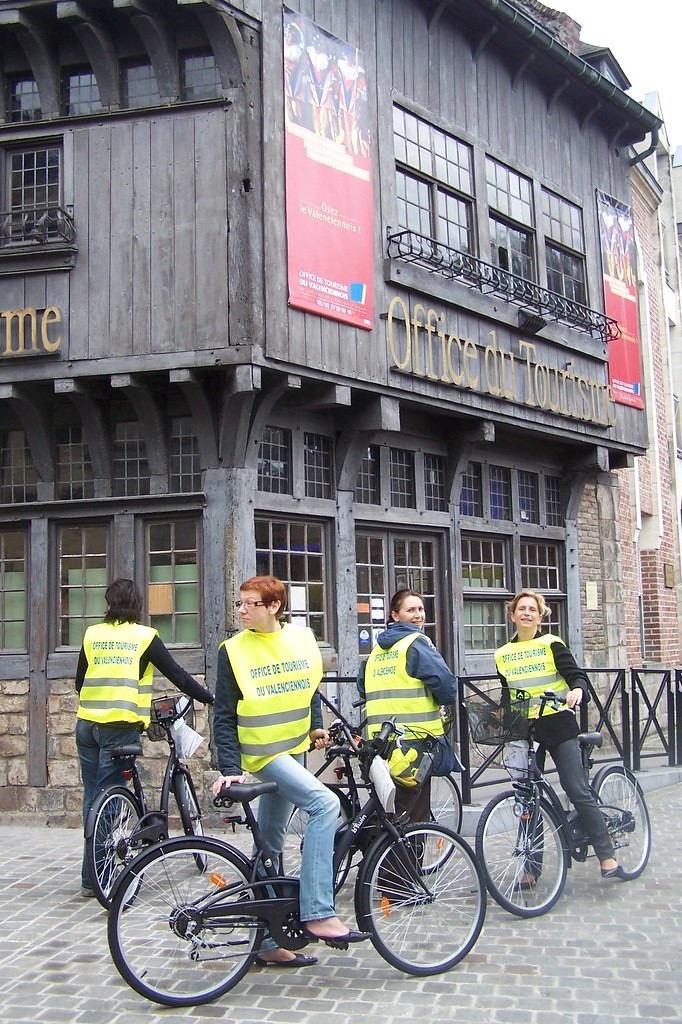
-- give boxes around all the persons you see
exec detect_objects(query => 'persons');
[76,578,215,897]
[356,589,458,904]
[212,576,373,965]
[494,591,622,890]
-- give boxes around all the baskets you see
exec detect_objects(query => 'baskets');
[146,695,194,741]
[463,686,531,746]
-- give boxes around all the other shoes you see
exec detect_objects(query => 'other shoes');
[80,884,96,896]
[382,887,427,905]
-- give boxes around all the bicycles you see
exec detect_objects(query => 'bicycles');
[103,717,487,1008]
[277,694,464,897]
[461,686,653,918]
[83,693,215,912]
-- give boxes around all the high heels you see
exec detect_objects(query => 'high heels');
[255,950,318,966]
[599,856,618,879]
[513,869,540,891]
[303,919,373,943]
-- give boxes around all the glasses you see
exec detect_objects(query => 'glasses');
[236,600,275,609]
